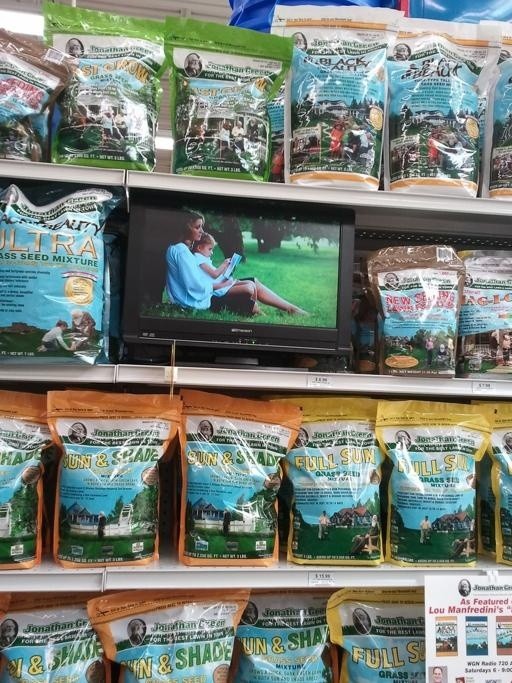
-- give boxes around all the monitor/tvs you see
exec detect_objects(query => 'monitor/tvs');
[122,187,355,373]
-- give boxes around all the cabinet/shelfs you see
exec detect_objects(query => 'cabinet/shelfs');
[2,163,127,683]
[104,170,512,682]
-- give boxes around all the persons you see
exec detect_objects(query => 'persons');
[161,210,311,316]
[195,233,241,289]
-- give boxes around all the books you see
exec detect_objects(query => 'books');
[223,253,241,278]
